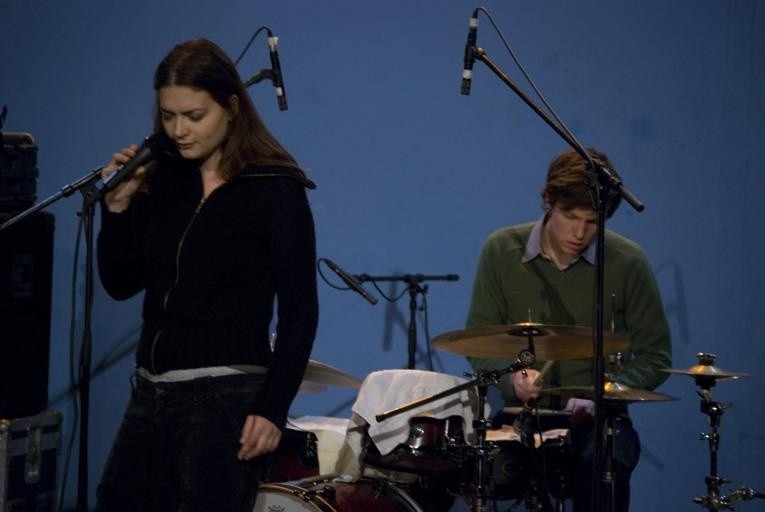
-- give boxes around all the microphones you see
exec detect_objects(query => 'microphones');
[460,9,480,96]
[514,397,538,434]
[266,31,289,112]
[86,131,169,204]
[323,257,378,306]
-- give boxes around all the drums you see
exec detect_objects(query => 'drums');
[466,426,567,500]
[363,369,493,474]
[267,416,366,481]
[253,465,421,512]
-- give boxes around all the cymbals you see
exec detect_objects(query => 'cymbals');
[268,343,363,394]
[535,381,680,403]
[658,365,755,380]
[431,322,632,361]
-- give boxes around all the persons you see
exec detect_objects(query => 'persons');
[94,36,322,512]
[447,146,674,510]
